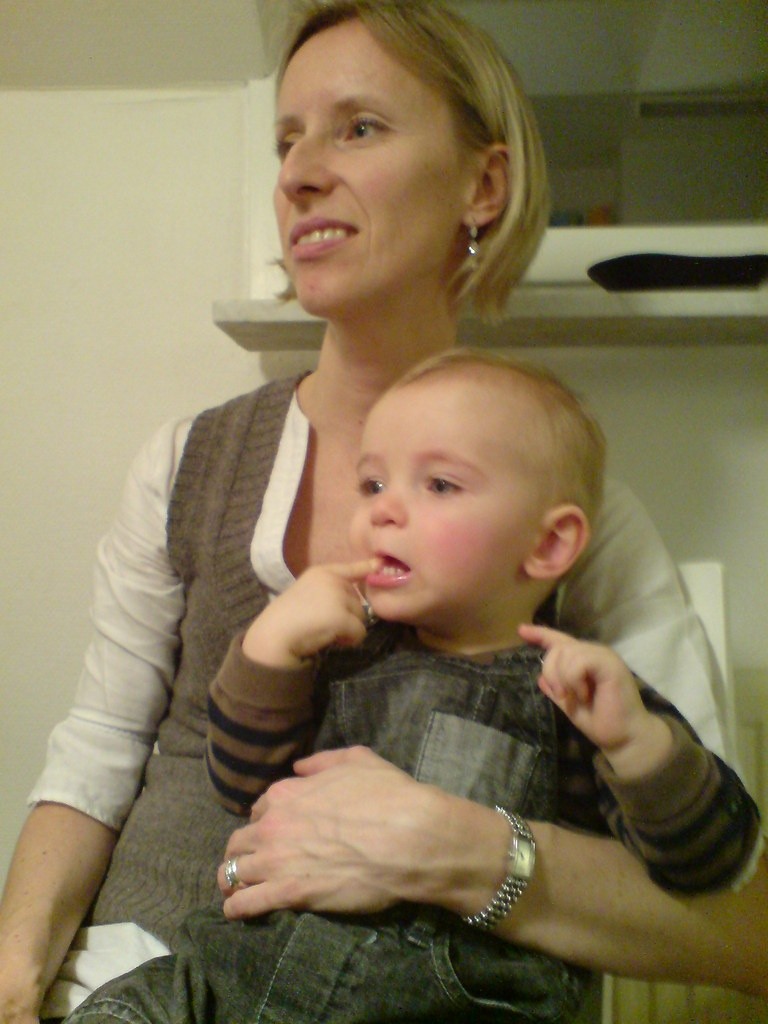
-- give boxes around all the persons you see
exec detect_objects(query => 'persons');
[0,0,768,1024]
[64,347,758,1024]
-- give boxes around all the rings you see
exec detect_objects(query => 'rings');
[225,859,246,888]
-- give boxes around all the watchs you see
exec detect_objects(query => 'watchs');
[462,803,538,928]
